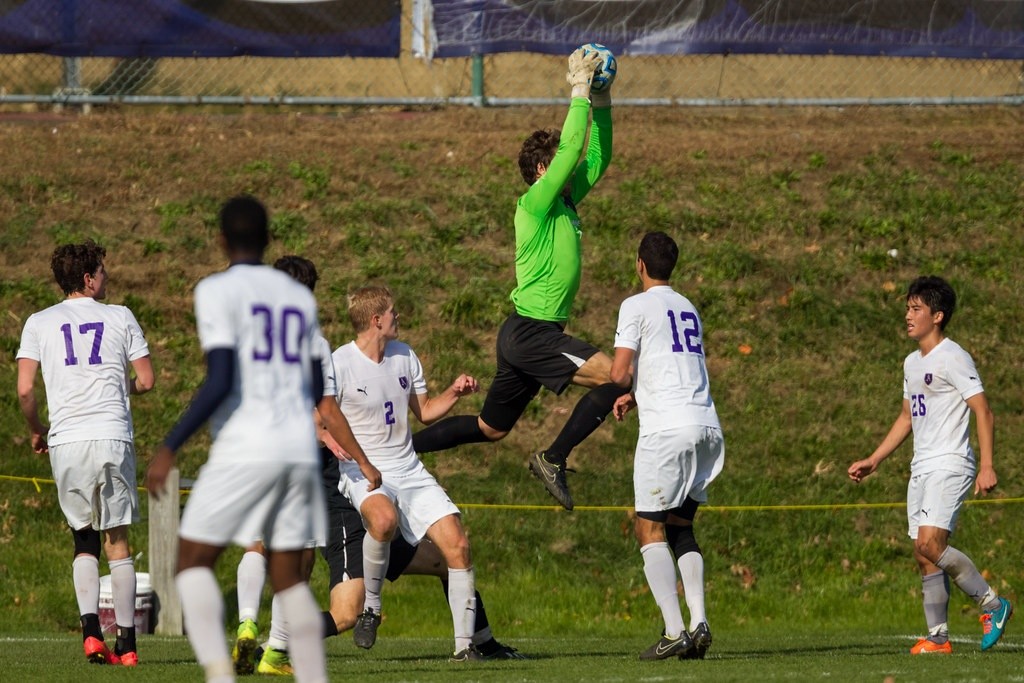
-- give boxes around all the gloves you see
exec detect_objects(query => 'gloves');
[589,84,611,106]
[565,47,603,98]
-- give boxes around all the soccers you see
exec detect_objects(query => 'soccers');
[569,43,617,92]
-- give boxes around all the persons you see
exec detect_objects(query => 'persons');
[414,47,633,510]
[14,240,155,664]
[610,231,724,663]
[847,274,1012,653]
[232,254,526,674]
[144,194,326,683]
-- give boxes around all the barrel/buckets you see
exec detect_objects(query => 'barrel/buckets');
[96,572,152,636]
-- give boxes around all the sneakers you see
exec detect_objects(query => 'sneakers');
[478,644,526,659]
[529,449,577,511]
[232,618,259,675]
[679,621,712,660]
[638,629,694,660]
[910,637,952,654]
[448,643,490,662]
[258,645,294,676]
[255,644,265,665]
[83,636,110,665]
[107,650,138,667]
[353,606,381,650]
[979,596,1013,651]
